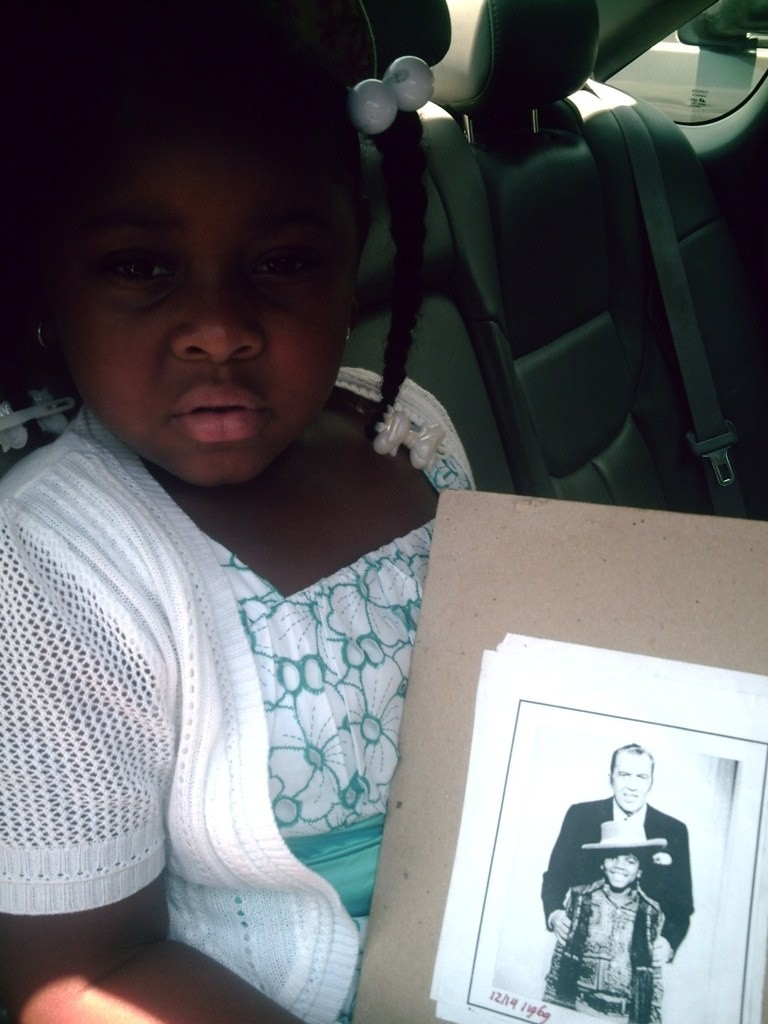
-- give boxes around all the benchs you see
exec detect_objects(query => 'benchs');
[340,0,768,523]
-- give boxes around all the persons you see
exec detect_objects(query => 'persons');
[0,1,478,1024]
[541,743,694,1022]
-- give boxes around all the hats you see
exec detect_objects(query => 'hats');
[581,818,667,863]
